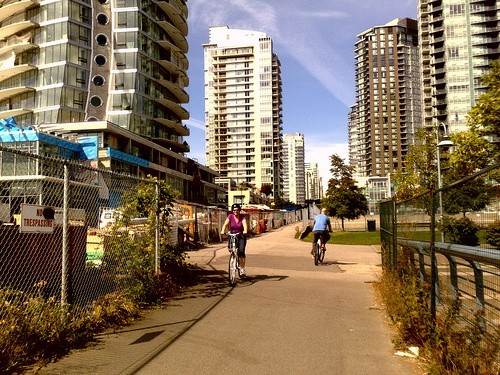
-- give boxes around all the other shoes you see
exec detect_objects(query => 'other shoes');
[321,246,326,251]
[240,269,246,275]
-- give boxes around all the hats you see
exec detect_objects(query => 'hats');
[232,204,241,211]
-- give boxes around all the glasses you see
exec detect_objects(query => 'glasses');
[233,210,239,211]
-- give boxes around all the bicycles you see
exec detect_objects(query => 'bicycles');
[224,231,245,285]
[313,231,330,266]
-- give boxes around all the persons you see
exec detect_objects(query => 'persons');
[221,203,248,277]
[310,207,333,255]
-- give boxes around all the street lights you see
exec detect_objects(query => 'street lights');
[435,122,457,243]
